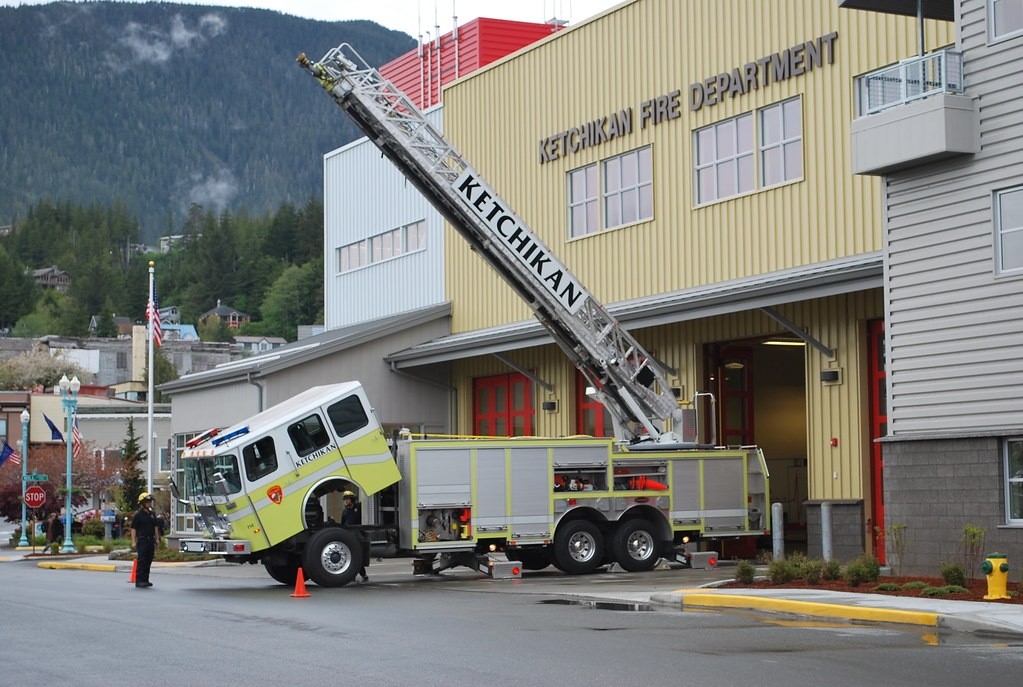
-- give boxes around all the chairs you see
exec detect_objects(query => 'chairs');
[295,424,317,454]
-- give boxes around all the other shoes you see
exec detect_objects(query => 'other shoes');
[145,581,152,586]
[360,576,368,580]
[136,581,147,587]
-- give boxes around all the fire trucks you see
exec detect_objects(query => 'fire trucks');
[166,45,772,588]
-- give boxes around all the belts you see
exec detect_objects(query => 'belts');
[139,536,153,539]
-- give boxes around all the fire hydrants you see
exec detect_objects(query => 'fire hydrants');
[981,550,1013,600]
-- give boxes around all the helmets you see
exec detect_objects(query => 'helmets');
[137,492,152,503]
[343,491,354,496]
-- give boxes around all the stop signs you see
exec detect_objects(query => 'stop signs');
[24,486,47,509]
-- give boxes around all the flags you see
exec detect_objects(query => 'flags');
[146,277,161,347]
[0,442,21,467]
[43,415,63,439]
[72,414,81,457]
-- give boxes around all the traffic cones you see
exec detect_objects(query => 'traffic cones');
[290,567,312,597]
[126,558,137,583]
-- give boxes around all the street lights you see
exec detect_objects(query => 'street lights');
[58,372,81,555]
[18,408,30,547]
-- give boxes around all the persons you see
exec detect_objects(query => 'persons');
[156,513,169,535]
[131,493,164,587]
[342,490,367,580]
[41,513,63,554]
